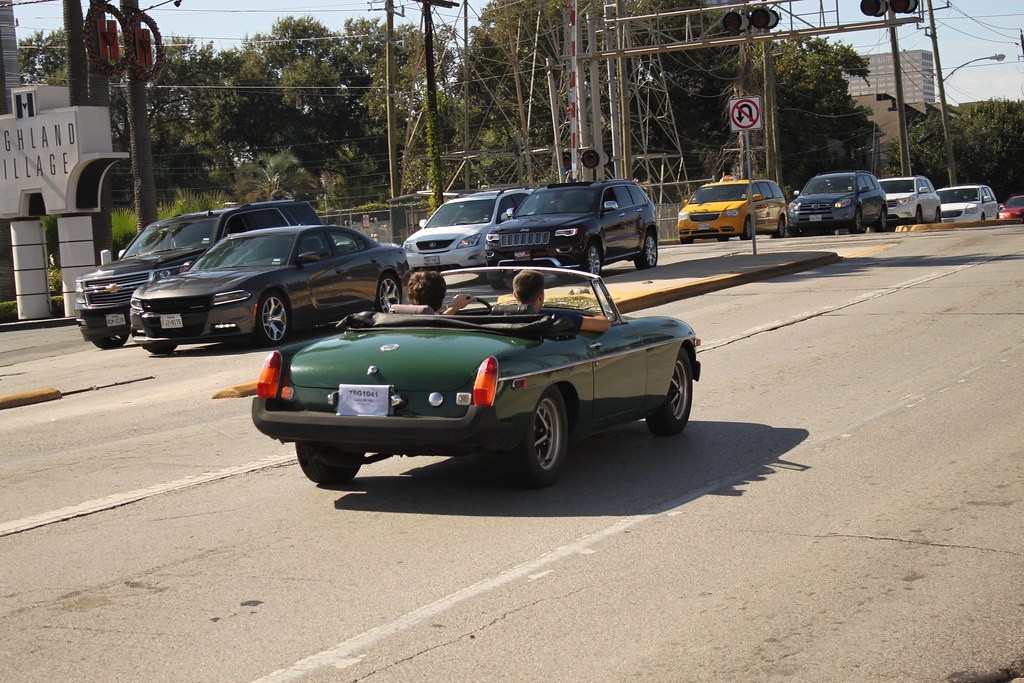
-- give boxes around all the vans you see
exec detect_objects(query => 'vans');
[677,176,788,243]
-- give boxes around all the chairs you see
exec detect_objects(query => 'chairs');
[491,304,538,314]
[390,302,435,315]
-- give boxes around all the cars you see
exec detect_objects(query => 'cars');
[130,225,410,358]
[934,184,999,225]
[998,195,1024,221]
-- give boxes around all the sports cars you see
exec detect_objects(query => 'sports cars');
[250,264,703,490]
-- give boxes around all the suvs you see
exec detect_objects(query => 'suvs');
[401,187,536,273]
[72,198,330,351]
[484,179,659,290]
[787,169,889,237]
[876,175,942,226]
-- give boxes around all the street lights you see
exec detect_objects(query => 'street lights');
[935,53,1006,186]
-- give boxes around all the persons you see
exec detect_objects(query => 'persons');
[407,270,475,315]
[582,192,601,211]
[513,270,609,332]
[222,223,232,237]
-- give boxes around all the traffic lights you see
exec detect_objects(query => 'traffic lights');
[890,0,918,14]
[750,8,779,29]
[723,12,751,32]
[582,150,599,168]
[860,0,888,16]
[554,151,572,171]
